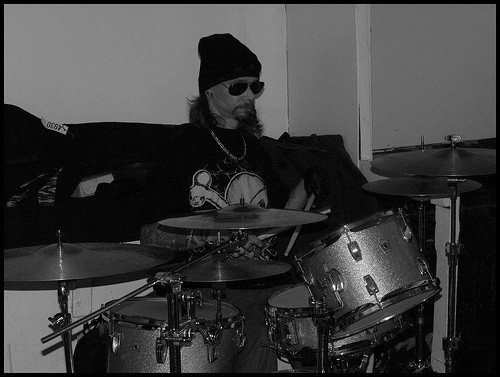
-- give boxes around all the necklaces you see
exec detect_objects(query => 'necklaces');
[208,126,247,161]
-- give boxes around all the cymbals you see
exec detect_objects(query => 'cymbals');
[158,203,328,230]
[154,256,292,283]
[370,147,496,176]
[363,176,483,197]
[4,241,175,282]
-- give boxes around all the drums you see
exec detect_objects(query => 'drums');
[101,297,246,373]
[264,280,413,361]
[292,206,442,340]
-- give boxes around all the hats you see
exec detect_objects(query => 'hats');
[196,33,263,94]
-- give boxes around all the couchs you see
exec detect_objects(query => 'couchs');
[5,105,345,243]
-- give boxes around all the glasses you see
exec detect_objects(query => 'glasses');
[220,78,266,97]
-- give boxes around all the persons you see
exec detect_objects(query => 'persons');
[145,34,308,373]
